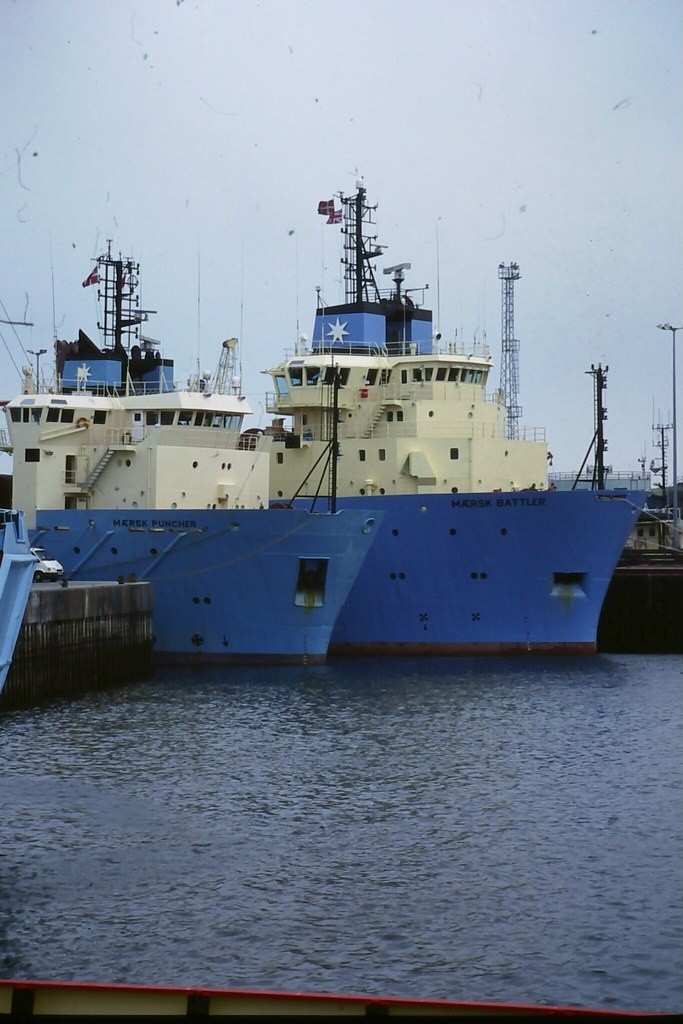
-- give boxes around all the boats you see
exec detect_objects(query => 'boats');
[0,177,681,704]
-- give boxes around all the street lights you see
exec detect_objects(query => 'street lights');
[657,322,683,550]
